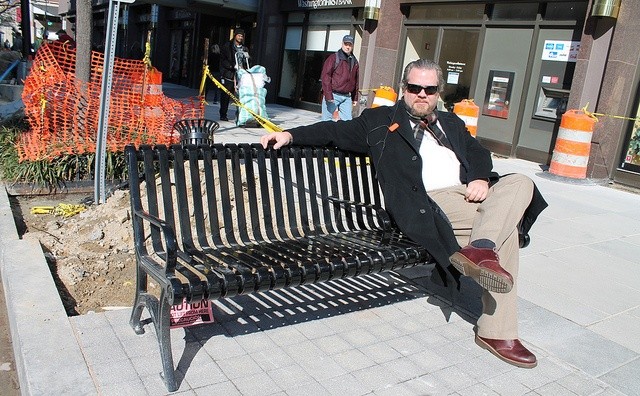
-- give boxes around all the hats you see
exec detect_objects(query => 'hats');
[343,35,354,44]
[233,29,244,38]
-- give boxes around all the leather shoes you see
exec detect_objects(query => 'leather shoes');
[475,334,537,369]
[448,243,513,294]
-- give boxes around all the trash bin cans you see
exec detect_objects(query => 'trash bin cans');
[174,117,219,159]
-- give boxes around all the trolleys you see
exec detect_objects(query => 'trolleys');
[234,50,269,128]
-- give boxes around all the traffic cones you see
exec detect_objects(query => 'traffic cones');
[371,86,399,109]
[535,108,594,187]
[452,98,480,141]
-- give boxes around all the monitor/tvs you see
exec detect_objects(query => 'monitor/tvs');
[447,71,460,85]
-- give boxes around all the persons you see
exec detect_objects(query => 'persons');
[11,32,22,50]
[260,58,549,369]
[320,34,359,121]
[219,29,252,121]
[56,29,76,42]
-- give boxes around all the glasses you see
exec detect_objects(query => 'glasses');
[405,84,438,95]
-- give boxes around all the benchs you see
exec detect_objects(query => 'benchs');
[124,145,488,392]
